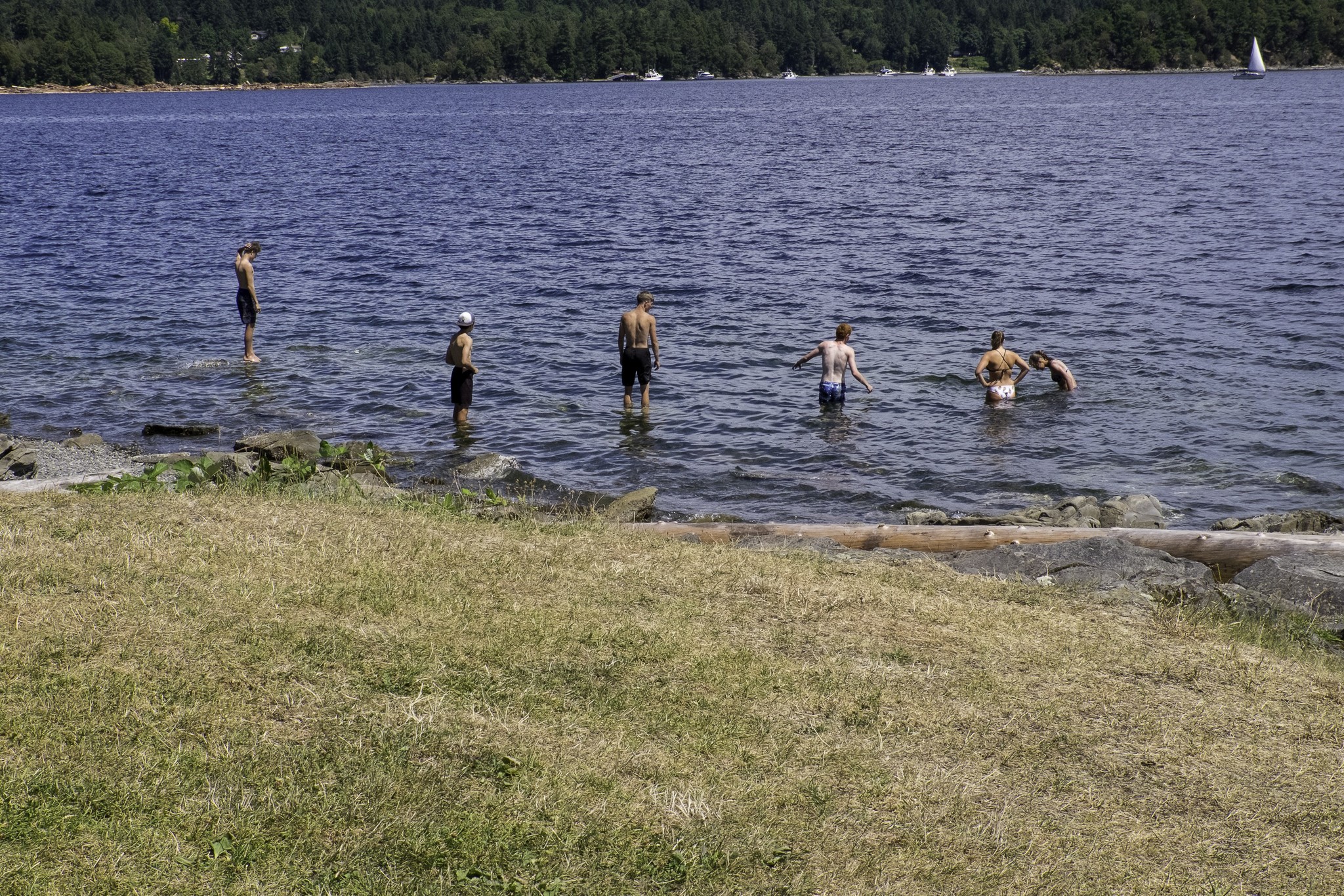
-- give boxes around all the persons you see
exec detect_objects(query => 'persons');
[1029,350,1077,391]
[792,323,873,408]
[617,291,660,403]
[234,242,263,363]
[975,331,1030,400]
[445,311,479,420]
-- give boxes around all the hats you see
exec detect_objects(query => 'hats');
[457,312,475,326]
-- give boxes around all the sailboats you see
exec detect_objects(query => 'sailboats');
[1232,36,1266,79]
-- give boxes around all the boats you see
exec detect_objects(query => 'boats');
[1010,68,1034,76]
[780,68,798,80]
[877,67,900,76]
[695,68,715,81]
[919,61,958,77]
[642,61,664,82]
